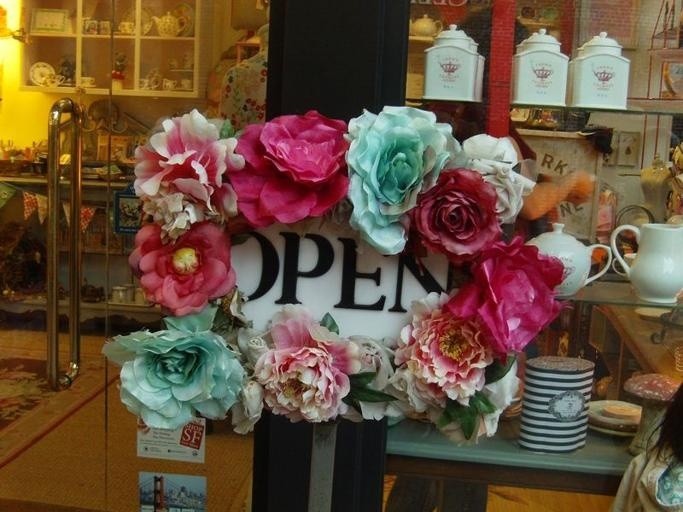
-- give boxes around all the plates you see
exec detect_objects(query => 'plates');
[588,399,642,438]
[29,62,56,86]
[82,85,97,87]
[635,307,671,323]
[121,6,153,36]
[114,31,132,35]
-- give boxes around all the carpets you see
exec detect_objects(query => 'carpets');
[3,331,131,469]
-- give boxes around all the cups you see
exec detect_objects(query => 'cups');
[81,77,95,85]
[118,22,134,32]
[45,74,65,87]
[612,254,638,281]
[516,28,562,54]
[434,23,479,53]
[112,283,149,305]
[577,32,622,58]
[162,79,177,90]
[181,79,193,89]
[32,154,47,174]
[83,19,115,35]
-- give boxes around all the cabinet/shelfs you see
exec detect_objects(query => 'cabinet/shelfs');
[386,81,683,478]
[14,0,215,98]
[2,174,161,327]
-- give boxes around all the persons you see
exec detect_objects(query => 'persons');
[421,6,598,241]
[604,380,683,512]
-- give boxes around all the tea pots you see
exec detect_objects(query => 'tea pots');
[523,223,613,297]
[610,223,683,304]
[409,14,443,37]
[151,11,187,37]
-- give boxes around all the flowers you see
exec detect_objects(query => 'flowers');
[107,113,549,432]
